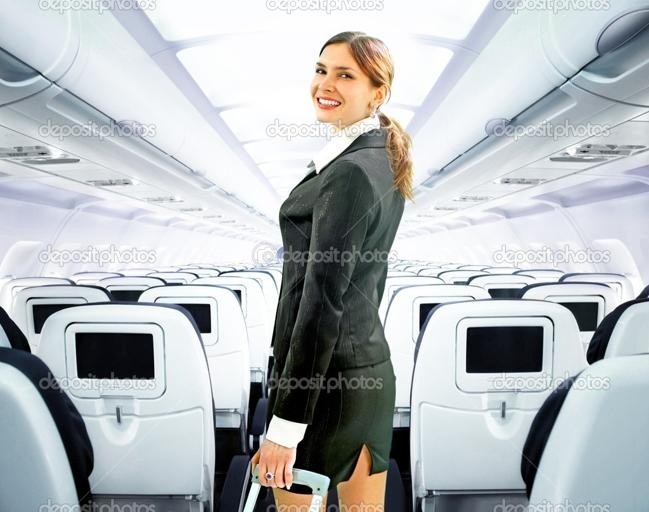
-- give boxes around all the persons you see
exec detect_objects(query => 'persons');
[248,27,416,512]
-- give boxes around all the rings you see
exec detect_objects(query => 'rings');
[265,472,275,480]
